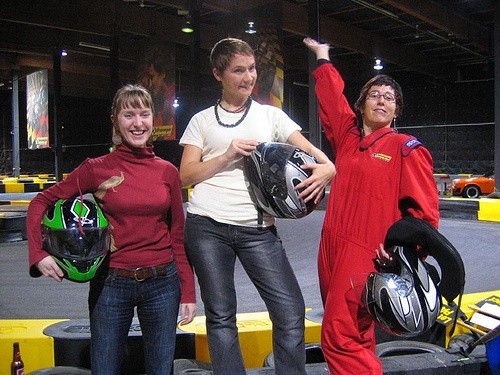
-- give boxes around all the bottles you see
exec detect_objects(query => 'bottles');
[11,342,25,375]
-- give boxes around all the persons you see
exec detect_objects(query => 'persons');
[303,37,439,375]
[27,84,197,375]
[178,37,337,375]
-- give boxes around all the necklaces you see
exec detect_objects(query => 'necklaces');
[215,95,252,128]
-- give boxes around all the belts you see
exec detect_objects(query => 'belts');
[102,264,169,281]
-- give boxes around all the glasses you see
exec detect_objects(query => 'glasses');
[364,91,398,103]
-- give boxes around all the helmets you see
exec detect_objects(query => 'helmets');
[40,197,113,283]
[243,141,328,219]
[365,244,442,337]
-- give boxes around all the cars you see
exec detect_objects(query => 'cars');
[448,169,495,198]
